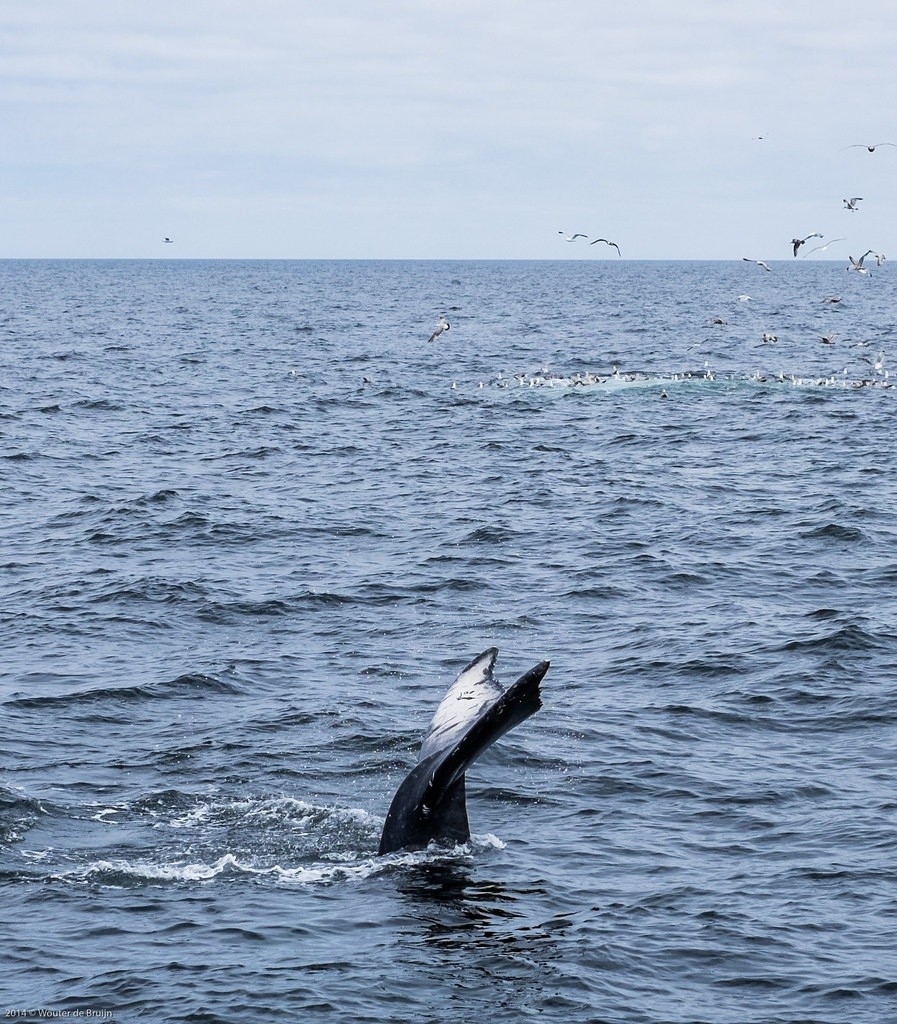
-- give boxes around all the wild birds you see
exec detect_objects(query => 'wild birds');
[160,132,896,398]
